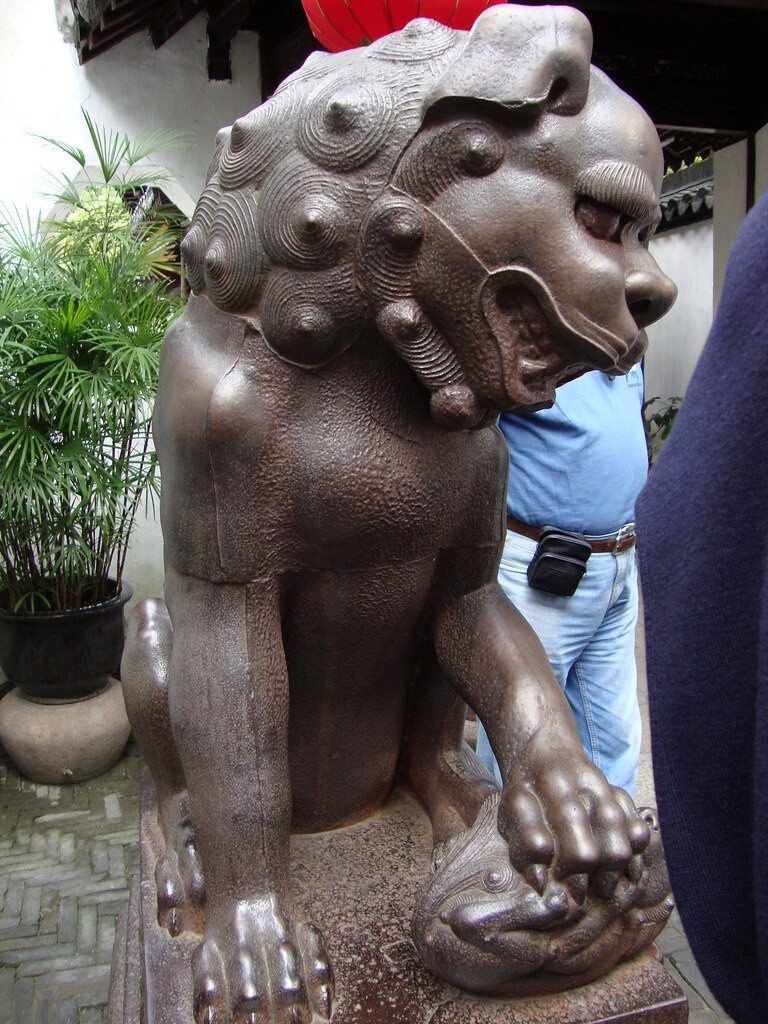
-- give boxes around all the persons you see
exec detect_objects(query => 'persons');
[476,356,651,796]
[633,195,768,1024]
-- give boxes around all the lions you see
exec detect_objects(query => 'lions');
[114,2,675,1024]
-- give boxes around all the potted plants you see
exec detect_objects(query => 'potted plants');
[0,106,194,706]
